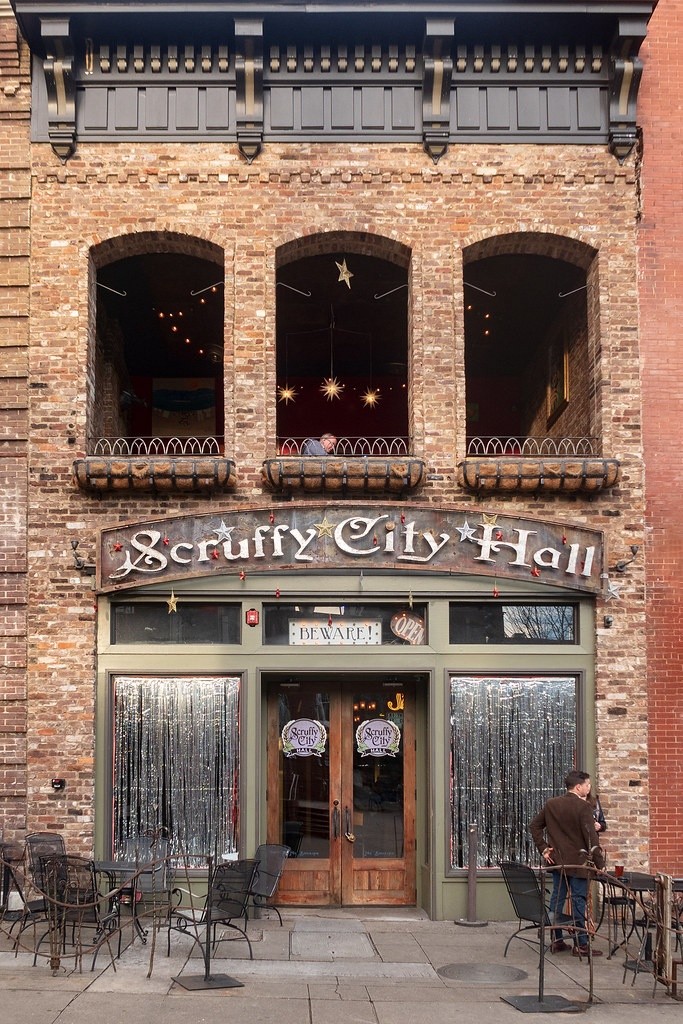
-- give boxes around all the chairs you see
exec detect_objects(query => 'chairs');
[1,832,682,1004]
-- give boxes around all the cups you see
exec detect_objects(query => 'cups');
[615,863,624,877]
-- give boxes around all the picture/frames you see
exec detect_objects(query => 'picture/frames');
[545,314,571,430]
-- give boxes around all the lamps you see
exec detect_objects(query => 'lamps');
[359,337,383,408]
[318,303,345,402]
[276,334,299,406]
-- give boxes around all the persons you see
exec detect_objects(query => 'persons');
[302,433,337,457]
[574,770,608,941]
[528,772,605,959]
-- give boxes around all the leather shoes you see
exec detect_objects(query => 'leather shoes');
[550,941,572,954]
[572,945,603,957]
